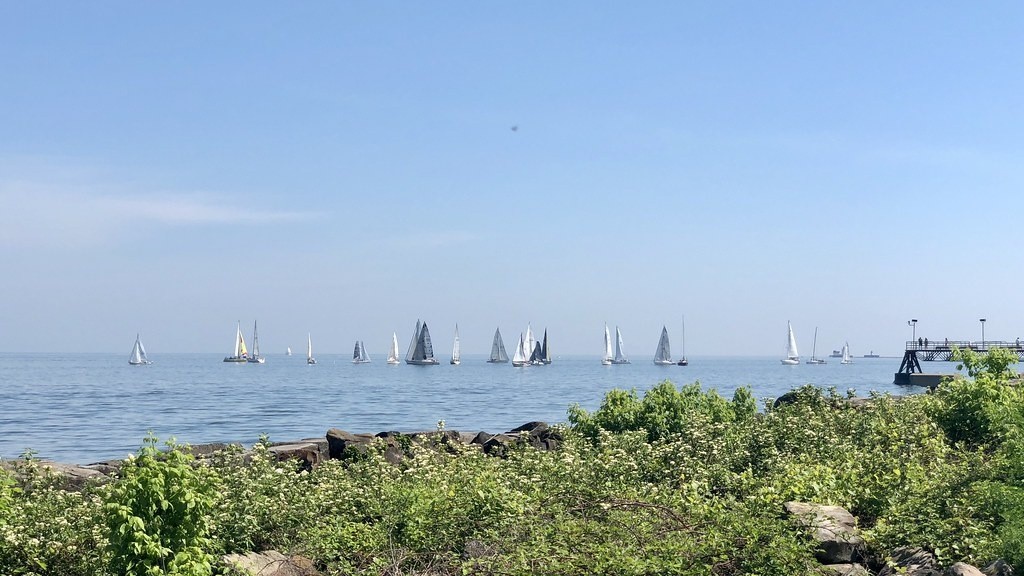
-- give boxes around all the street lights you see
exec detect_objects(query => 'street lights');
[911,319,917,350]
[980,319,986,350]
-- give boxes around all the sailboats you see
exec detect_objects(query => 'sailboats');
[249,320,266,364]
[450,323,460,365]
[841,342,850,364]
[780,320,799,365]
[223,320,249,362]
[404,318,439,365]
[387,332,400,364]
[601,322,614,365]
[286,347,291,354]
[127,333,152,365]
[678,315,687,365]
[614,326,630,364]
[352,340,370,363]
[487,328,509,363]
[653,324,674,365]
[512,322,552,367]
[806,327,826,365]
[307,331,316,365]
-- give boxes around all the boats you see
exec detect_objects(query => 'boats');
[830,350,853,357]
[864,351,879,358]
